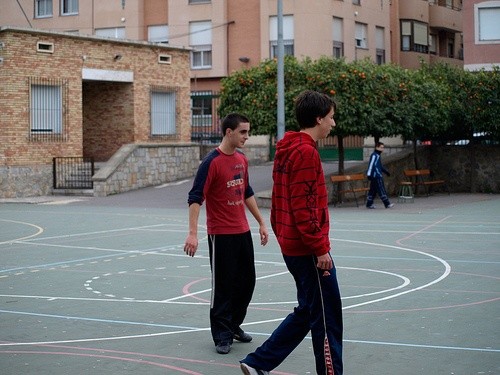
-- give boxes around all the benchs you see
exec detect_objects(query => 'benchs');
[403,169,445,198]
[330,174,371,208]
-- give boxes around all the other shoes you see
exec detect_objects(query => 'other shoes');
[384,204,393,208]
[234,326,252,343]
[366,205,376,209]
[216,341,231,354]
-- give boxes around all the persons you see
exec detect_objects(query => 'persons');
[366,142,395,209]
[237,90,344,375]
[183,113,269,354]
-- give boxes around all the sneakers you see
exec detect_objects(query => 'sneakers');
[241,362,269,375]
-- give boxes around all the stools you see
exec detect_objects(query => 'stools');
[398,182,414,203]
